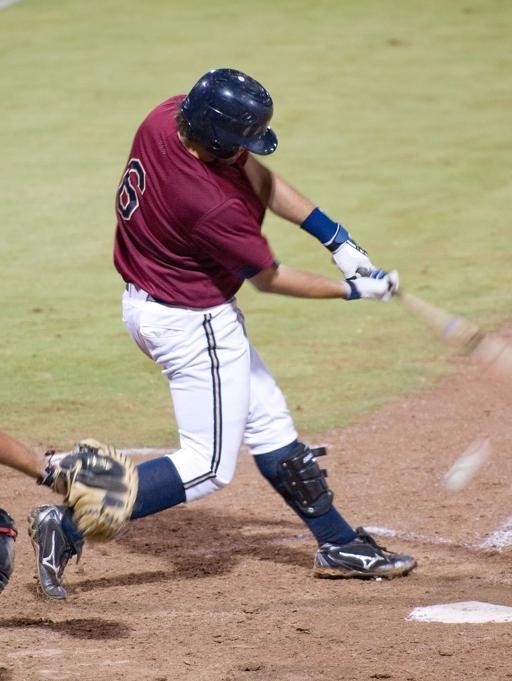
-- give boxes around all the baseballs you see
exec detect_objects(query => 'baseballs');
[443,468,471,493]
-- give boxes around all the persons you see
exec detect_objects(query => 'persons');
[25,63,424,601]
[1,431,139,595]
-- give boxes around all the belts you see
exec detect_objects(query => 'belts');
[125,282,162,301]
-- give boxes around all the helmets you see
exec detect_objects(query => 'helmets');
[180,68,278,158]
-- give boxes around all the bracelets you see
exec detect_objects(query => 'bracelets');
[299,207,339,245]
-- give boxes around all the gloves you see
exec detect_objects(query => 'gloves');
[323,222,400,300]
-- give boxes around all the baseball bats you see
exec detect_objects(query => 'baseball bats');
[358,264,512,398]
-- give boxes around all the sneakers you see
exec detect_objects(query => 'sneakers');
[27,504,84,601]
[311,526,417,579]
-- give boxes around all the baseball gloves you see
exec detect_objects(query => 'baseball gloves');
[43,438,139,540]
